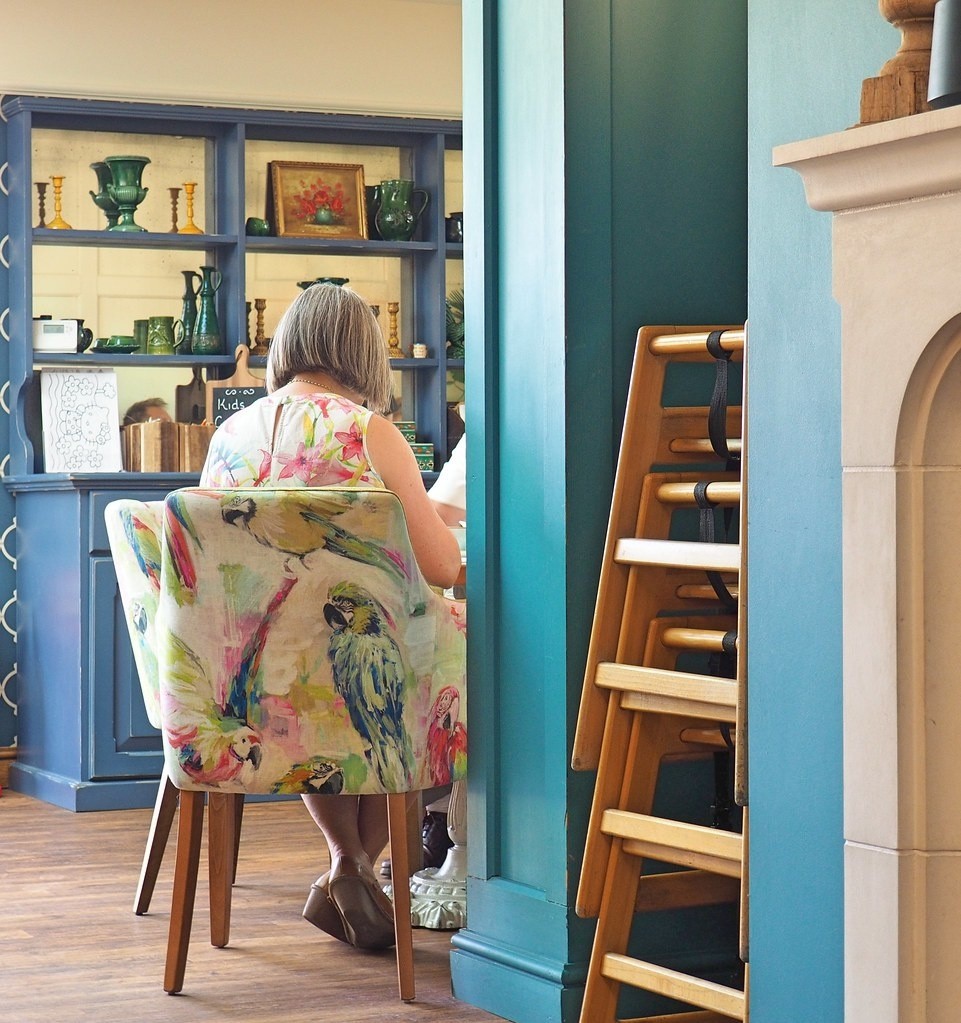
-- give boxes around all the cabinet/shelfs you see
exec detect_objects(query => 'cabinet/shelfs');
[0,94,464,813]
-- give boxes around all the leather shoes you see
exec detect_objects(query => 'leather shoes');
[380,812,455,877]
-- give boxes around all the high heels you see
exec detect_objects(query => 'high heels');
[301,868,351,945]
[328,856,396,951]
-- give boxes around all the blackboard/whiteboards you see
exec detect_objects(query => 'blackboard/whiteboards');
[205,378,269,430]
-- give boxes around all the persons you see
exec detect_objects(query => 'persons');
[380,432,467,878]
[199,284,462,950]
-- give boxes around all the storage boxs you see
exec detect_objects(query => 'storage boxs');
[392,421,434,472]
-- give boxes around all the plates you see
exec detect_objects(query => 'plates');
[89,345,141,354]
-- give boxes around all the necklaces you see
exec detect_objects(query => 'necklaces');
[286,380,335,394]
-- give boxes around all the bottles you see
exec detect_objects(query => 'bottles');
[176,271,203,355]
[191,266,223,355]
[375,180,429,241]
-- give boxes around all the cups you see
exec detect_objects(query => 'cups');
[133,319,148,354]
[147,316,185,354]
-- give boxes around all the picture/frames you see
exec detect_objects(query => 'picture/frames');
[271,161,367,240]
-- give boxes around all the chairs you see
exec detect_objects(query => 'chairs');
[104,484,465,1004]
